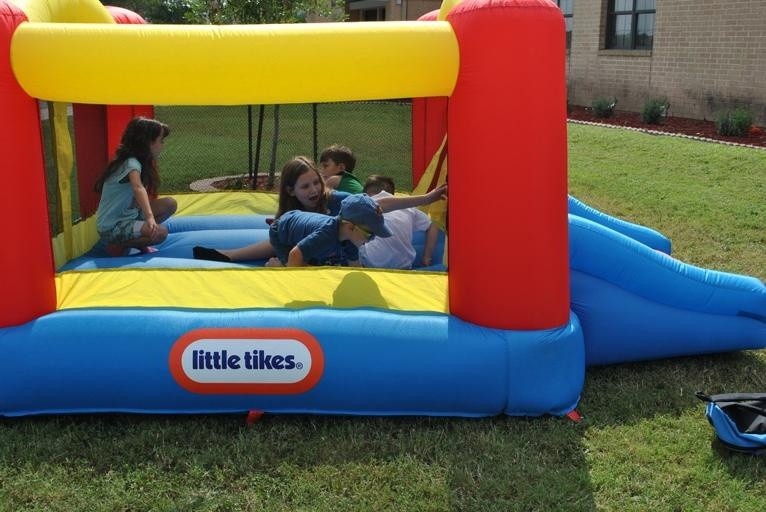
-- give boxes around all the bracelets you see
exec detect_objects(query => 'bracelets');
[420,258,432,261]
[420,263,432,267]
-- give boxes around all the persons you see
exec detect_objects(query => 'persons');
[264,192,394,267]
[265,143,362,225]
[358,174,438,270]
[93,116,176,256]
[192,157,446,263]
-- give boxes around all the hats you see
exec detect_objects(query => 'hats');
[338,192,393,239]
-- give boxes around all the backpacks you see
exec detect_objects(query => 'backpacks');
[690,388,766,459]
